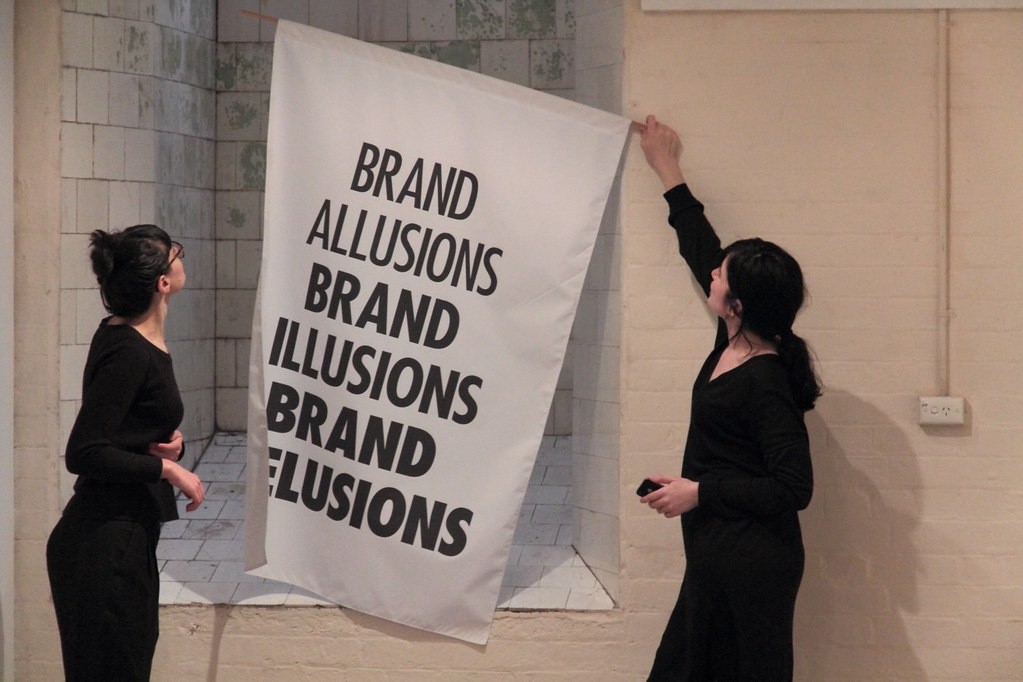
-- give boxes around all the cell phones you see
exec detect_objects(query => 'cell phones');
[636,478,665,497]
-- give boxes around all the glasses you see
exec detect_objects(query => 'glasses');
[156,241,185,292]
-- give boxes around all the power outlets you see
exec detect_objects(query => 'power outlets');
[919,396,964,425]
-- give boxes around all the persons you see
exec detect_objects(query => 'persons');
[629,113,825,682]
[46,222,205,682]
